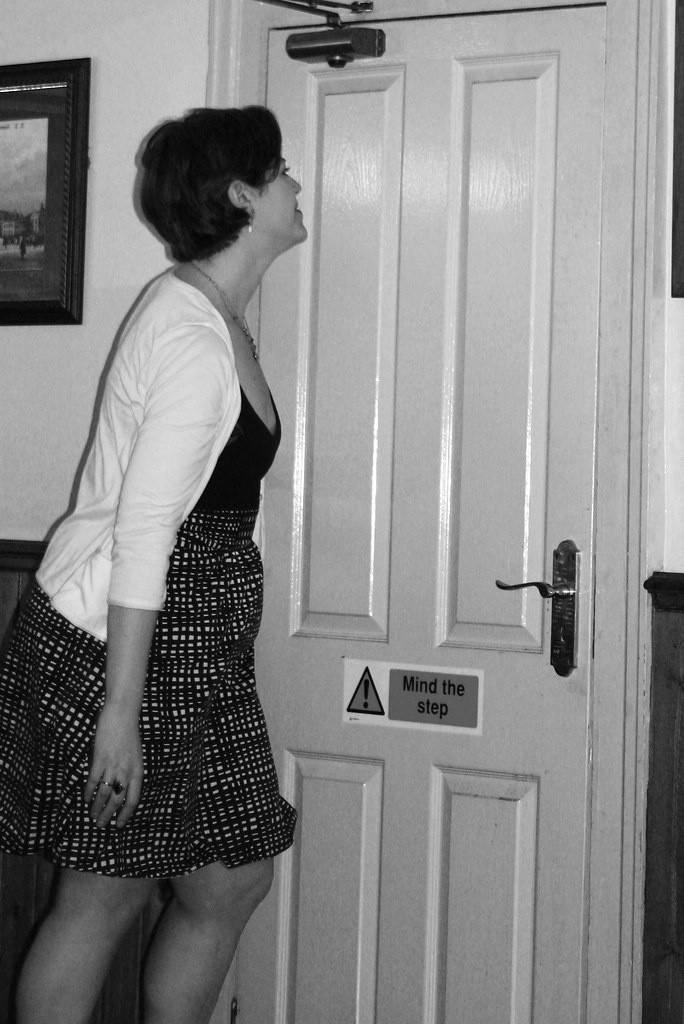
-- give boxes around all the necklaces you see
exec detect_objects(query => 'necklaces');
[190,261,258,361]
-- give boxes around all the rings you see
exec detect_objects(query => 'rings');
[114,781,127,795]
[101,780,114,787]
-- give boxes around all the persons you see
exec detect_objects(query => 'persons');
[0,106,309,1024]
[3,234,43,260]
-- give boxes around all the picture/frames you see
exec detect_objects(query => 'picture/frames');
[0,56,93,326]
